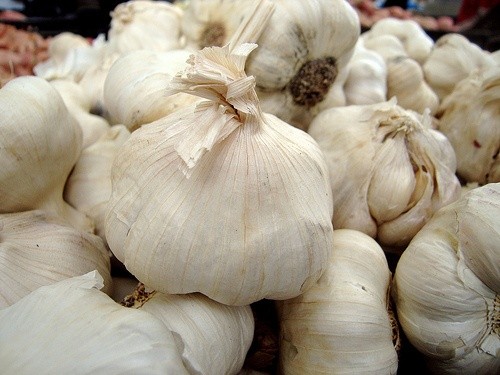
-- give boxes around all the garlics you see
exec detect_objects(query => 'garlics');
[0,0,500,375]
